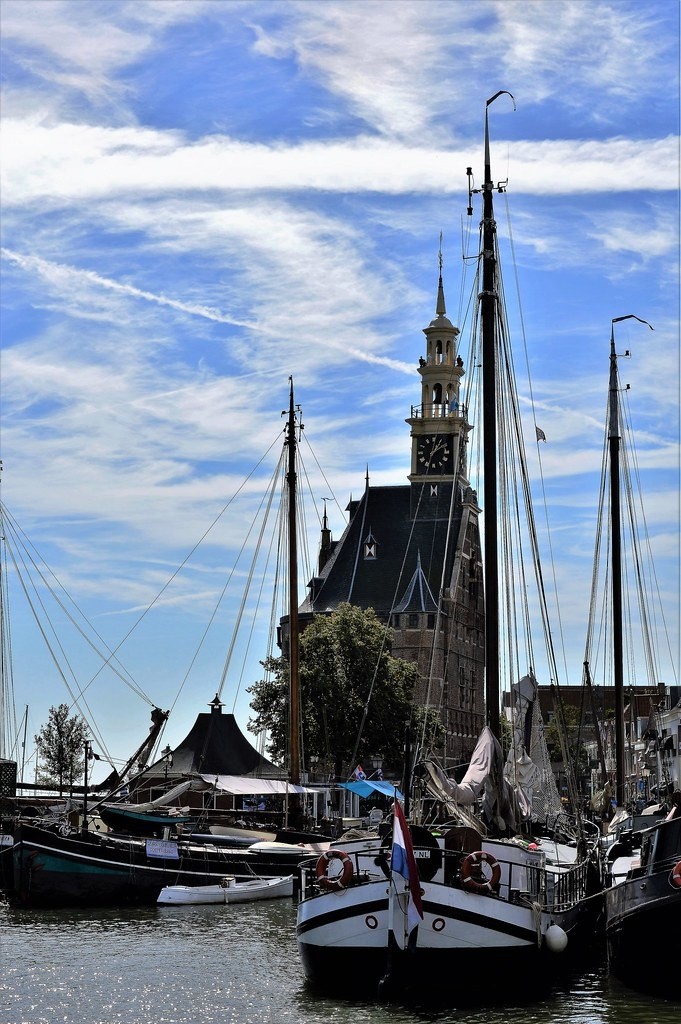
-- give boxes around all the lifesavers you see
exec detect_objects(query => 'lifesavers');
[315,849,353,892]
[672,860,681,885]
[462,851,502,894]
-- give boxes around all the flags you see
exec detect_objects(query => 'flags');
[388,802,424,950]
[536,427,546,442]
[377,768,384,780]
[355,766,366,782]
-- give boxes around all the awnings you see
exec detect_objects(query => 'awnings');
[200,775,319,795]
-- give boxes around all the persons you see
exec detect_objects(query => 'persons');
[600,780,613,823]
[118,780,130,796]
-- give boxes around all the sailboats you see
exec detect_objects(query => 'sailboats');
[582,318,681,986]
[290,87,608,993]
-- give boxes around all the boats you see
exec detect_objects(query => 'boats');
[1,371,323,906]
